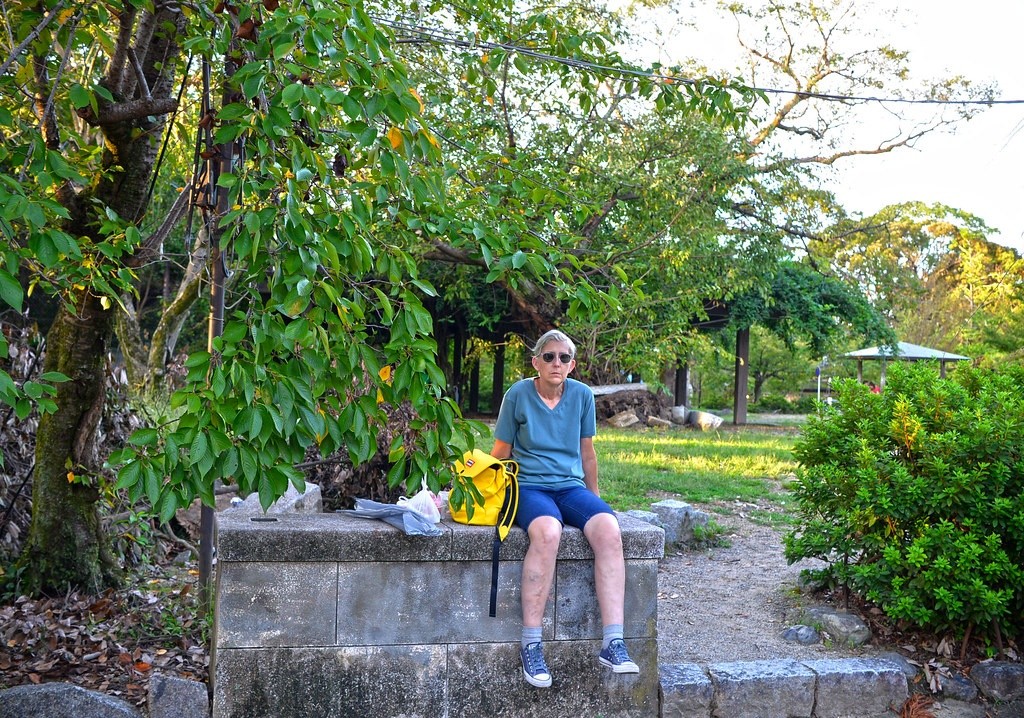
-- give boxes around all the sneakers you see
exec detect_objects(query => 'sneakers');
[519,642,552,687]
[599,638,639,674]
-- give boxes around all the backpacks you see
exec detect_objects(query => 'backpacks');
[448,448,519,543]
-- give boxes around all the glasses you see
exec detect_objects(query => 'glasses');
[541,352,572,363]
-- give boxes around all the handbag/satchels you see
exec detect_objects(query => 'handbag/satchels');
[397,472,440,524]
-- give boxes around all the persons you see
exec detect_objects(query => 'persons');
[863,379,881,395]
[491,329,640,688]
